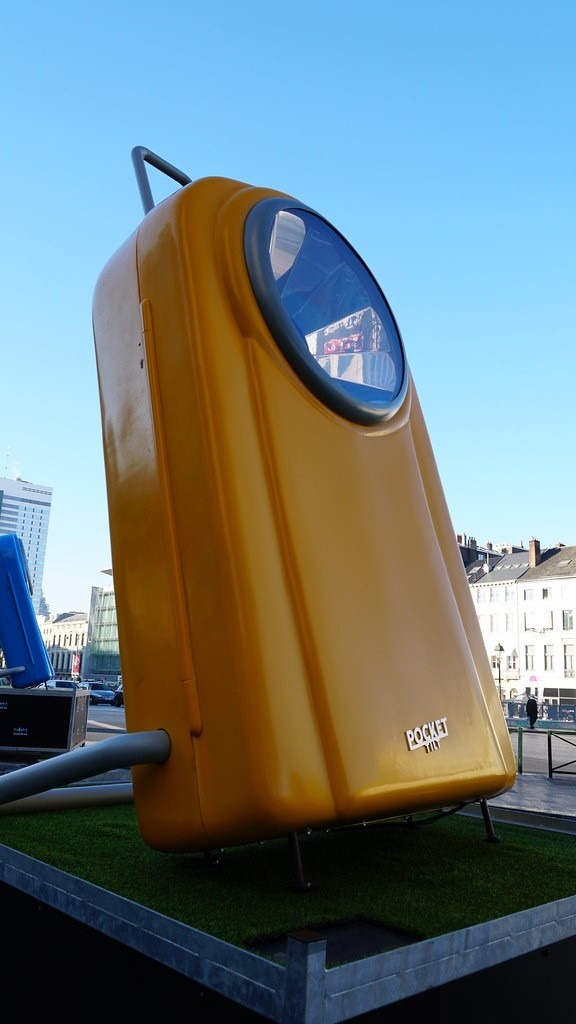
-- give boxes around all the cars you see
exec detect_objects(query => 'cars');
[77,682,115,705]
[37,680,124,707]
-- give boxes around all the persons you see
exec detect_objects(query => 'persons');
[525,694,538,729]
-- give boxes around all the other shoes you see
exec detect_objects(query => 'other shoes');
[530,727,535,729]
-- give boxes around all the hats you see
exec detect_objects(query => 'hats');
[529,694,538,700]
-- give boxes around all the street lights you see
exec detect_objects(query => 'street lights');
[494,643,505,700]
